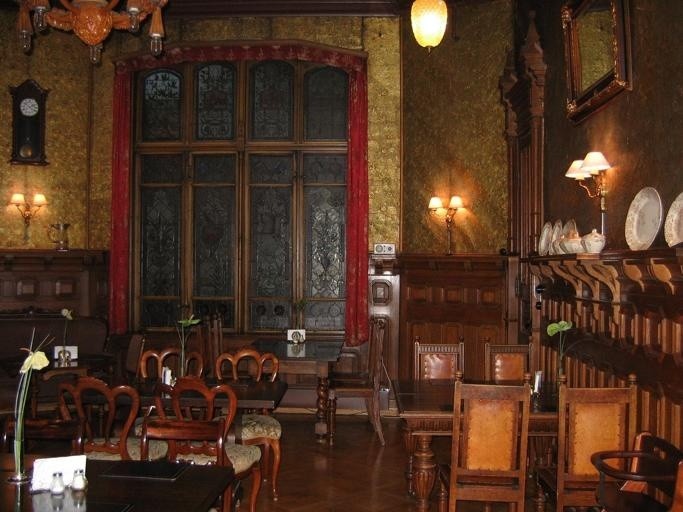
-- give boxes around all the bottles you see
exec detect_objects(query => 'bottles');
[70,469,88,490]
[49,472,64,494]
[521,368,548,414]
[50,491,85,510]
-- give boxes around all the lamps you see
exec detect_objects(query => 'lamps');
[11,192,49,225]
[412,0,452,54]
[429,195,464,227]
[17,2,166,67]
[568,152,616,212]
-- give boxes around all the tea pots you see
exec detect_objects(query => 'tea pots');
[48,222,70,252]
[552,228,606,254]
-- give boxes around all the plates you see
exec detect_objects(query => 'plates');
[663,193,682,248]
[625,186,664,251]
[538,219,577,257]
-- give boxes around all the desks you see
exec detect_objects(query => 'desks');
[386,376,578,508]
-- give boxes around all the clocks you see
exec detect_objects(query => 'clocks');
[7,77,51,167]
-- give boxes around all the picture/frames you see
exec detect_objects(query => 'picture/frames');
[557,6,633,124]
